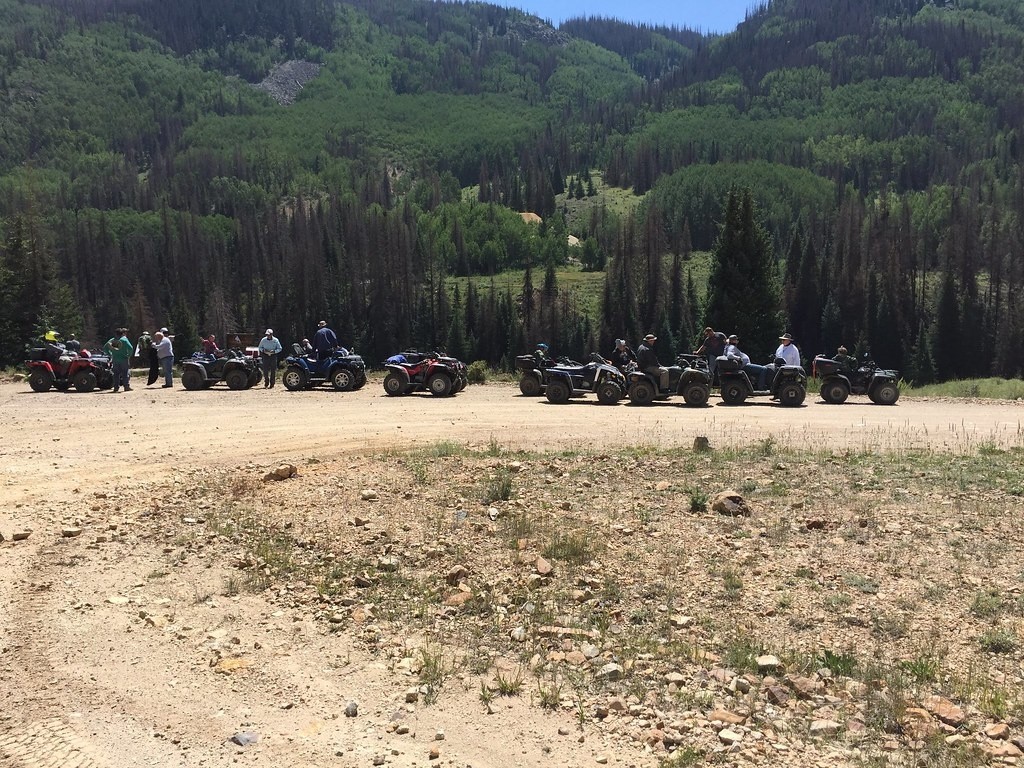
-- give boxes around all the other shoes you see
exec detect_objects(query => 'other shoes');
[270,383,274,389]
[162,384,172,388]
[124,388,133,391]
[854,379,865,386]
[114,386,119,391]
[659,389,671,394]
[770,395,781,400]
[265,381,269,388]
[542,380,549,385]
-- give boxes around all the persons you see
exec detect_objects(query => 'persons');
[612,339,638,368]
[259,329,282,389]
[200,335,220,354]
[535,343,548,361]
[138,331,152,367]
[693,327,727,372]
[776,333,800,366]
[46,331,77,370]
[70,334,80,351]
[312,321,338,377]
[833,347,855,370]
[637,334,670,393]
[724,335,767,390]
[151,331,175,388]
[104,328,134,392]
[303,339,313,354]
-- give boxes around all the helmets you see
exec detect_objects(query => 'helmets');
[45,331,61,343]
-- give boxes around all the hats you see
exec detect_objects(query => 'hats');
[317,320,328,327]
[112,327,123,336]
[615,339,626,346]
[160,328,169,334]
[140,332,151,336]
[121,328,129,332]
[778,333,794,341]
[643,334,657,341]
[108,340,123,350]
[536,344,548,349]
[264,329,274,335]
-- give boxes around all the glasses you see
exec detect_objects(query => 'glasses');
[734,338,739,341]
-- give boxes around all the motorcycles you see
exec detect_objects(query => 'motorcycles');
[716,356,807,406]
[178,345,264,391]
[626,354,713,406]
[513,354,587,399]
[544,350,627,406]
[278,344,368,391]
[814,356,900,404]
[381,347,470,398]
[24,348,130,393]
